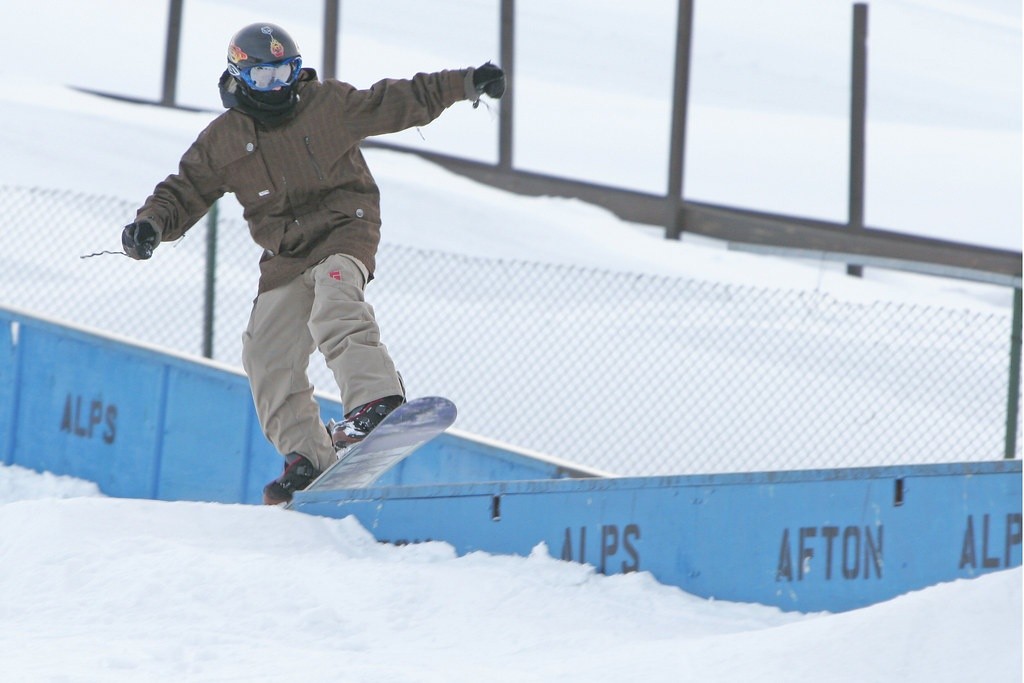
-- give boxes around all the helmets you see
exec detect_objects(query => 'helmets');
[227,23,301,104]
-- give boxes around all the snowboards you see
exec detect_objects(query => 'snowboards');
[283,396,457,511]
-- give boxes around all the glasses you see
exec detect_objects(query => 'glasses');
[240,57,302,91]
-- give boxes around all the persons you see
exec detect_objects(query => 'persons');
[120,20,506,507]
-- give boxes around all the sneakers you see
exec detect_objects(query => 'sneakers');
[263,452,324,505]
[331,394,404,449]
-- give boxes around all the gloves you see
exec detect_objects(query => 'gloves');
[122,222,156,260]
[473,62,505,99]
